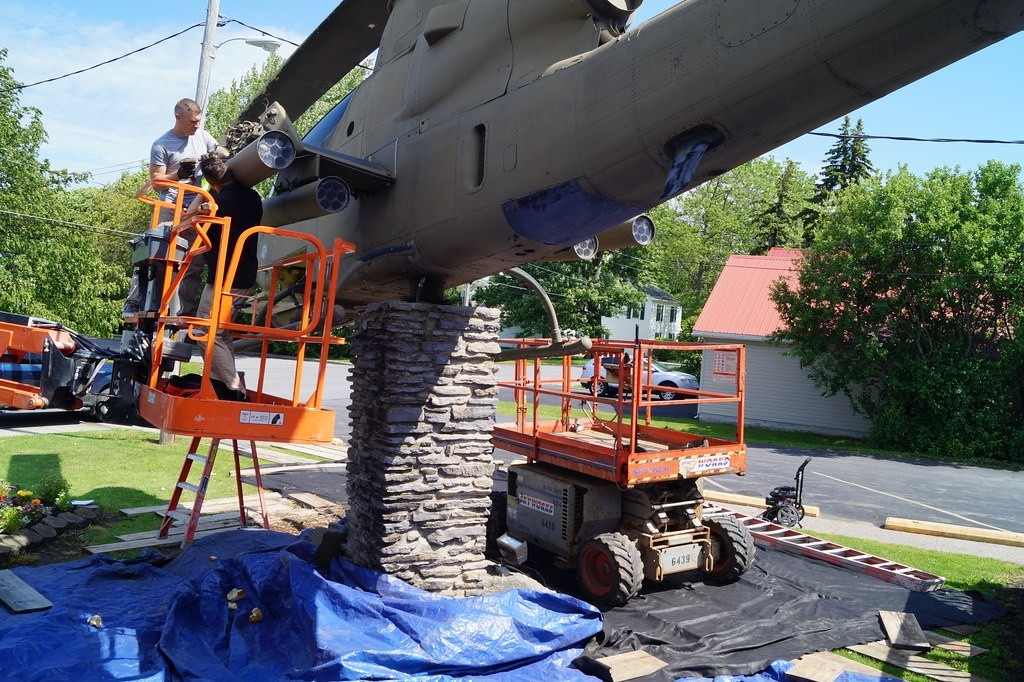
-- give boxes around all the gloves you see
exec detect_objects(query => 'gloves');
[177,164,195,179]
[201,176,212,192]
[201,151,215,160]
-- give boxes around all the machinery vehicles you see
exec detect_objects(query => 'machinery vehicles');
[489,334,754,604]
[1,178,356,445]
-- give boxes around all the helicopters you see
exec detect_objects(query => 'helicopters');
[211,0,1024,331]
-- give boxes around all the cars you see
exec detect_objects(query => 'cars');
[1,351,113,422]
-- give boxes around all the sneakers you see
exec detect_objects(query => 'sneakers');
[122,303,139,317]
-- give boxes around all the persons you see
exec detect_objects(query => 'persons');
[122,98,229,318]
[195,155,263,401]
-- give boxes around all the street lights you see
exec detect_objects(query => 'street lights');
[196,36,281,128]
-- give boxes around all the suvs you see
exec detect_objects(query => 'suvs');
[580,356,696,400]
